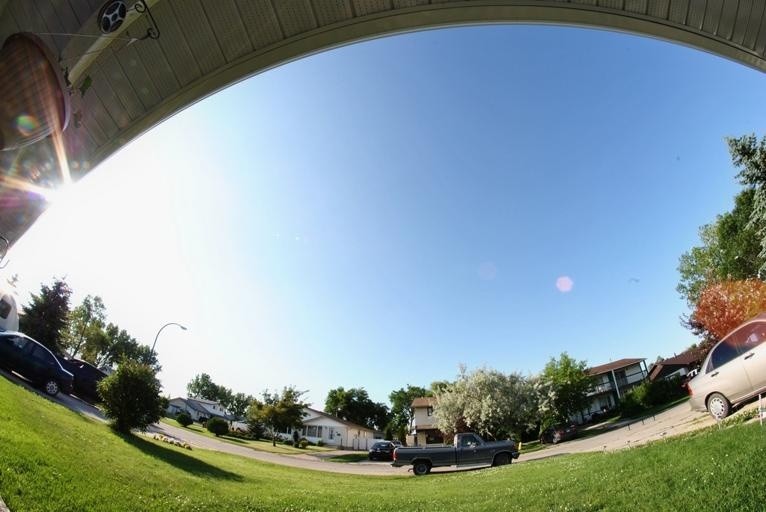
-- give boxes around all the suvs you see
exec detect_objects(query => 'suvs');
[56,351,110,407]
[663,372,689,393]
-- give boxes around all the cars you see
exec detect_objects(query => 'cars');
[368,436,407,465]
[538,420,578,446]
[685,368,699,378]
[0,329,75,398]
[687,316,766,422]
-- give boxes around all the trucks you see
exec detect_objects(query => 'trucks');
[0,283,19,334]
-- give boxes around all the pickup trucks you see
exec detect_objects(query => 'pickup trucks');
[392,430,522,476]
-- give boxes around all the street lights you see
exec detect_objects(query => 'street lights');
[147,321,188,376]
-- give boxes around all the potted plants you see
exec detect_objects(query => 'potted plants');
[0,26,96,156]
